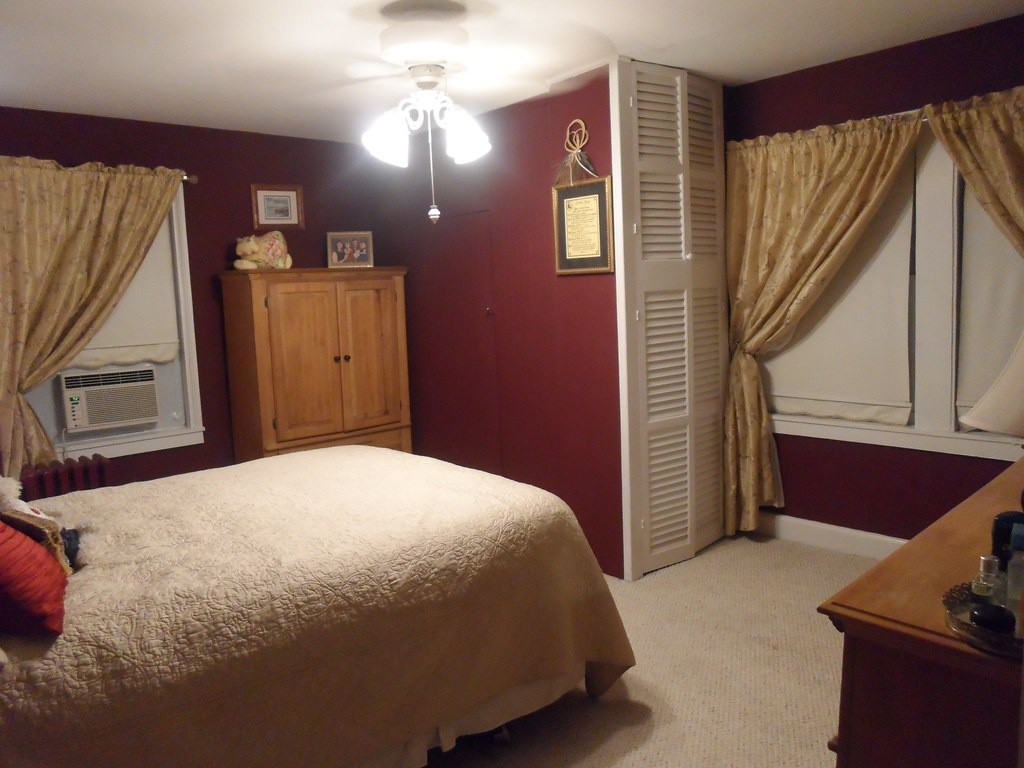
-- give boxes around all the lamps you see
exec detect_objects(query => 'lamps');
[358,87,492,170]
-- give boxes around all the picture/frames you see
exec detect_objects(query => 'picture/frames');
[551,176,616,276]
[326,230,374,268]
[250,182,306,231]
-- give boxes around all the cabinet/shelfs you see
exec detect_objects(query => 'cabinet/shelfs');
[218,267,415,464]
[818,451,1024,768]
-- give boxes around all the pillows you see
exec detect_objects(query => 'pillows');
[0,476,79,639]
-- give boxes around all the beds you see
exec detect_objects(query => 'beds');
[0,444,636,768]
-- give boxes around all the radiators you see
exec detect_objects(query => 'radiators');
[22,453,111,501]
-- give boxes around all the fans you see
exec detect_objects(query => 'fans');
[233,0,619,108]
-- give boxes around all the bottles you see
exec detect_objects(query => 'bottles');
[969,553,1008,607]
